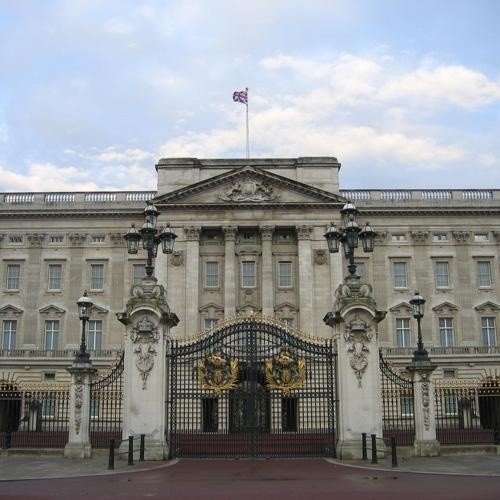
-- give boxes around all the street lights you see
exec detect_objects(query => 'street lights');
[325,200,379,279]
[409,292,431,361]
[122,199,178,279]
[69,291,96,369]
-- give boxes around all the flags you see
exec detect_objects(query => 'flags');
[232,88,251,106]
